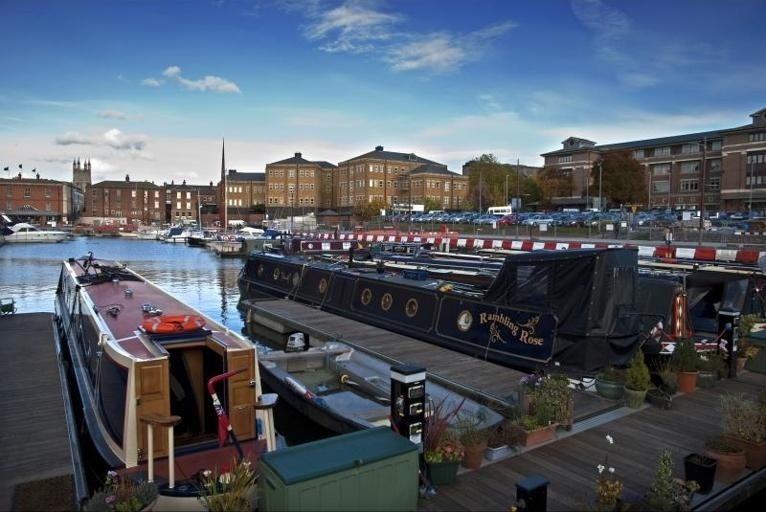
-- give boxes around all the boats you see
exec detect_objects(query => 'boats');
[52,252,295,512]
[258,336,505,455]
[117,222,263,260]
[5,223,69,245]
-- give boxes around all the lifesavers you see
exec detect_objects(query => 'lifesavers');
[143,314,206,334]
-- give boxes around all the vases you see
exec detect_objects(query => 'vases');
[427,462,459,483]
[520,393,536,415]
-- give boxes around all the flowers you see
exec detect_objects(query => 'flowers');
[423,391,467,462]
[519,373,547,393]
[194,453,263,511]
[593,430,626,506]
[86,469,158,512]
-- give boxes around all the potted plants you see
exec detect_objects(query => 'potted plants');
[623,339,766,511]
[483,419,526,460]
[506,412,561,446]
[594,368,625,399]
[452,402,487,469]
